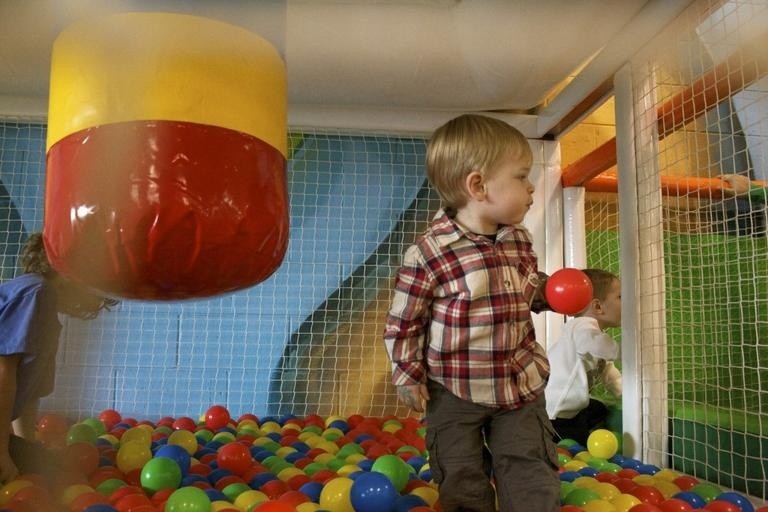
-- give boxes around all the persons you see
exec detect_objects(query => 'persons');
[715,171,768,206]
[381,114,591,512]
[0,232,122,486]
[543,267,622,442]
